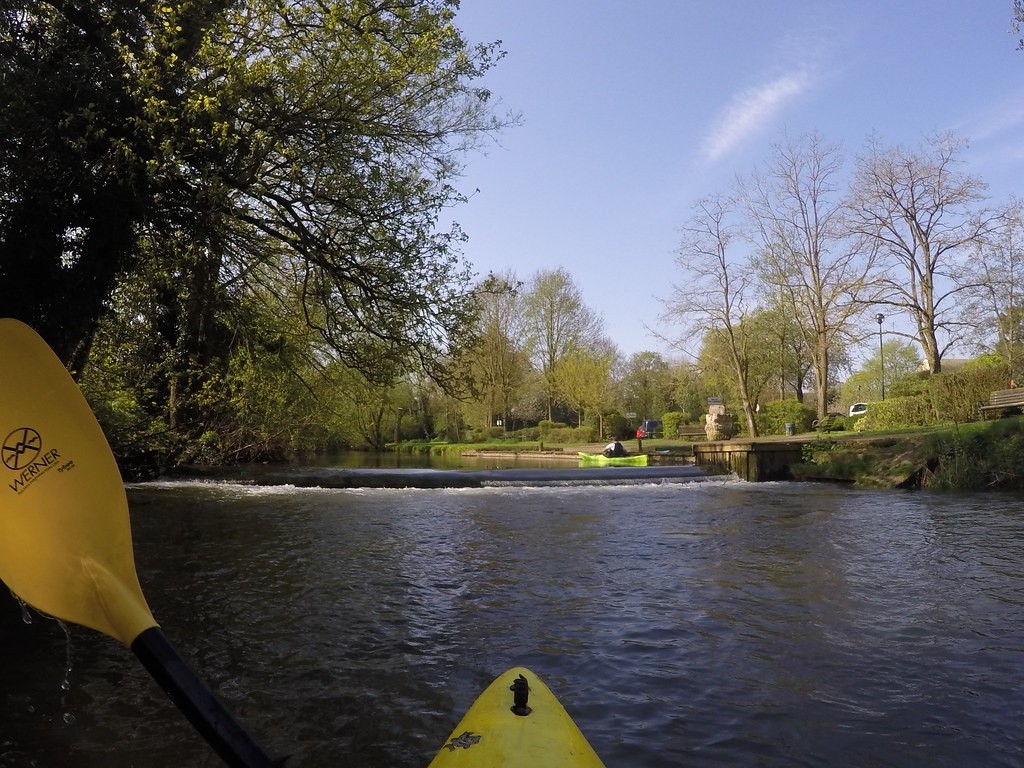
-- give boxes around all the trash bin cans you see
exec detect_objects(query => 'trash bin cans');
[785,423,796,436]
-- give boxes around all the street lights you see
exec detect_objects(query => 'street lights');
[875,313,885,401]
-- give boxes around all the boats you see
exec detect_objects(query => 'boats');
[578,461,648,469]
[578,452,649,463]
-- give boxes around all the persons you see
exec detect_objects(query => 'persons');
[602,435,629,458]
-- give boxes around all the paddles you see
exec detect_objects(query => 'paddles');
[2,314,277,768]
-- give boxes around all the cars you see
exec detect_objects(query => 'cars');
[849,403,867,418]
[636,420,665,439]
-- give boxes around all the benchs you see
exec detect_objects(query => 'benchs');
[976,387,1024,422]
[812,414,836,433]
[678,425,707,441]
